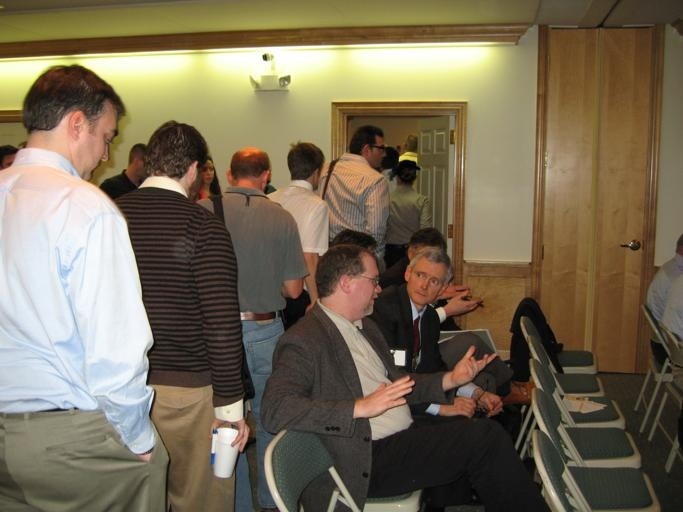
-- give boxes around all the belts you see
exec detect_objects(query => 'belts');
[240,311,275,321]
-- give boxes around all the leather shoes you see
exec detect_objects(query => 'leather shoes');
[498,380,535,405]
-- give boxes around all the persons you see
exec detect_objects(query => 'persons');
[193,146,310,510]
[262,246,543,512]
[97,121,249,511]
[2,145,19,168]
[646,234,682,366]
[0,63,170,512]
[333,229,530,442]
[265,143,329,327]
[660,267,682,339]
[318,125,388,260]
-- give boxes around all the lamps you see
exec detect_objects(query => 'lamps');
[250,53,290,93]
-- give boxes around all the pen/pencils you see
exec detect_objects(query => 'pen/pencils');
[463,296,485,308]
[210,427,218,464]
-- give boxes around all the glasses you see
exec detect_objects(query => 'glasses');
[413,316,420,354]
[362,275,378,287]
[370,145,385,151]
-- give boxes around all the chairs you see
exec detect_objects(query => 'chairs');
[635,300,683,474]
[513,316,662,511]
[264,425,421,512]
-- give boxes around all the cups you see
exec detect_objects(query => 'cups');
[213,427,240,479]
